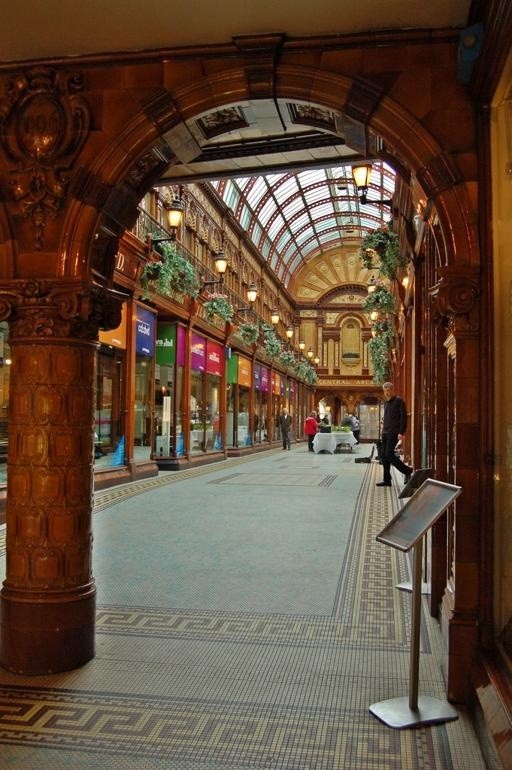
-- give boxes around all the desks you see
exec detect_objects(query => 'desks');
[312,430,359,454]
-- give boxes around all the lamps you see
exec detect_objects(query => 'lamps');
[146,195,183,247]
[354,161,397,209]
[366,277,392,297]
[204,247,320,368]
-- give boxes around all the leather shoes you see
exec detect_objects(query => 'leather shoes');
[376,481,392,486]
[404,468,413,484]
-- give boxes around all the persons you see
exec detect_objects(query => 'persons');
[376,382,413,486]
[316,413,361,445]
[305,410,318,452]
[279,408,293,450]
[254,413,258,442]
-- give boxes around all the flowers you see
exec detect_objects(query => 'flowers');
[357,220,411,280]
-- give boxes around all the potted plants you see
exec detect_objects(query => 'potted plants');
[369,318,394,338]
[164,244,198,303]
[138,260,166,301]
[362,283,396,314]
